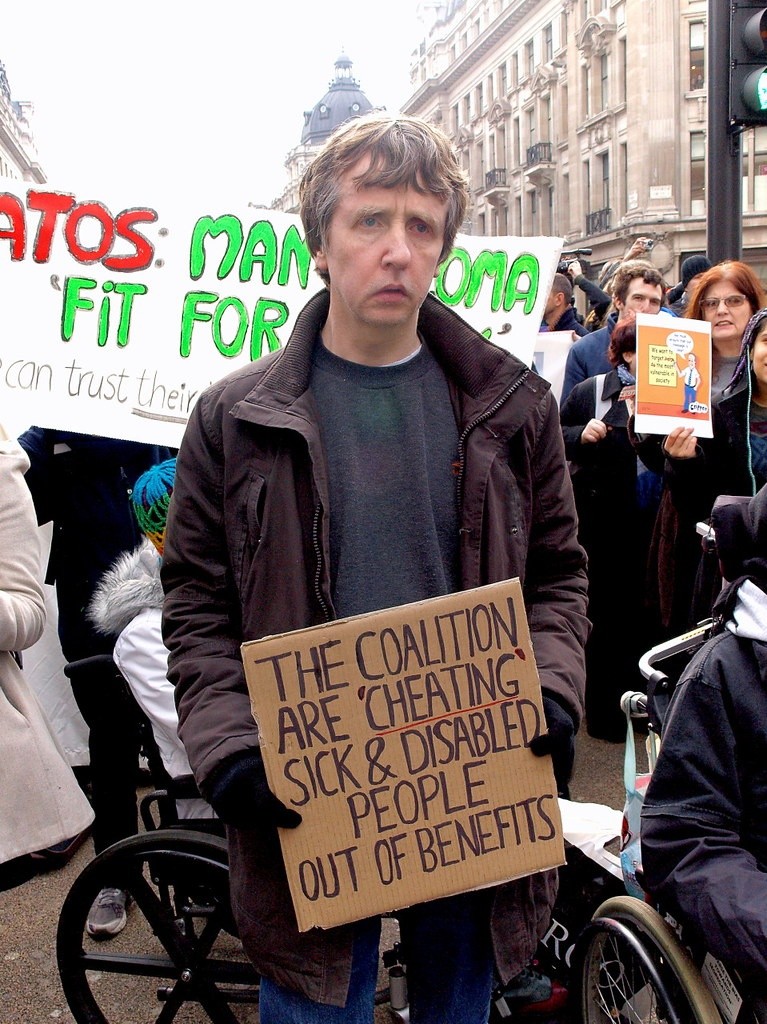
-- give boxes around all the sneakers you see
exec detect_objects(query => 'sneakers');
[87,887,134,937]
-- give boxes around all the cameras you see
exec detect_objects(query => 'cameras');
[641,239,653,251]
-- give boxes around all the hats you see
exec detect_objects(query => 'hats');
[681,254,711,288]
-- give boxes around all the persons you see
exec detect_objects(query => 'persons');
[159,112,595,1024]
[0,420,237,938]
[526,237,767,1024]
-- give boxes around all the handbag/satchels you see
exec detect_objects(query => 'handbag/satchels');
[620,695,658,909]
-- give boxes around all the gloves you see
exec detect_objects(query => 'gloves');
[530,691,580,790]
[202,745,304,880]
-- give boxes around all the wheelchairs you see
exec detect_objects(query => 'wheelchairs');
[565,616,767,1023]
[55,656,416,1024]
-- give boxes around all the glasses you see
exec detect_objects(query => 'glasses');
[698,294,746,313]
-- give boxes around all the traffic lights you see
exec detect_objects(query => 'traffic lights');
[729,0,767,124]
